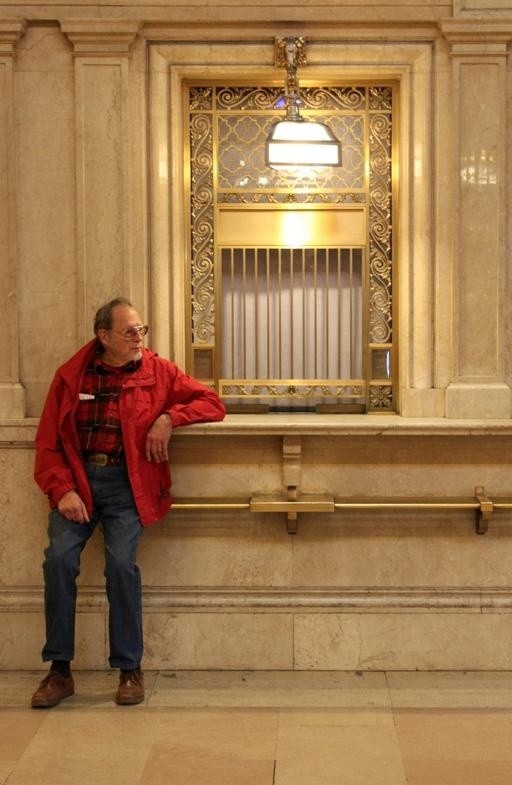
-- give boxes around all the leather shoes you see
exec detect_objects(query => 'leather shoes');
[115,671,145,705]
[31,671,75,708]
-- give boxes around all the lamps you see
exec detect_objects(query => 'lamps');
[264,34,342,174]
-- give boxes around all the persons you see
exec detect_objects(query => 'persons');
[31,297,226,708]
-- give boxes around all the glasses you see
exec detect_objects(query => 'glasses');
[106,325,149,341]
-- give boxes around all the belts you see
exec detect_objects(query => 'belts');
[82,452,125,467]
